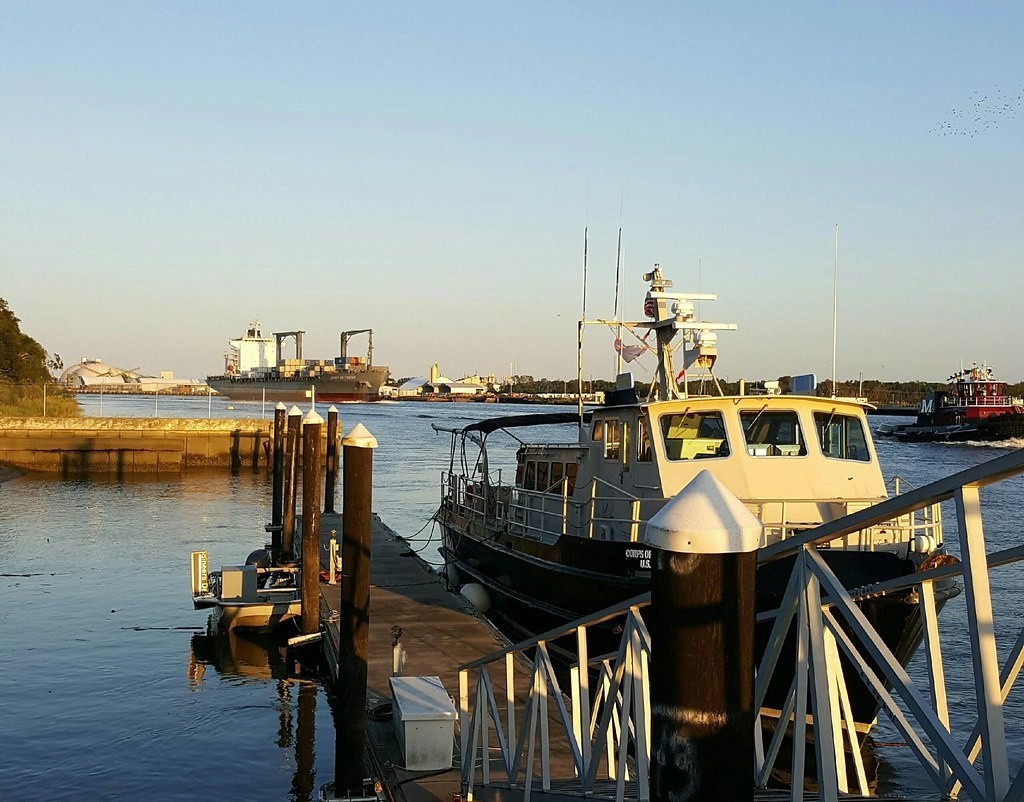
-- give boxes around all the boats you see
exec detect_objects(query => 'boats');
[874,358,1024,443]
[428,227,967,754]
[188,548,302,633]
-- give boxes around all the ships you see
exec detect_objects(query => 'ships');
[204,317,392,401]
